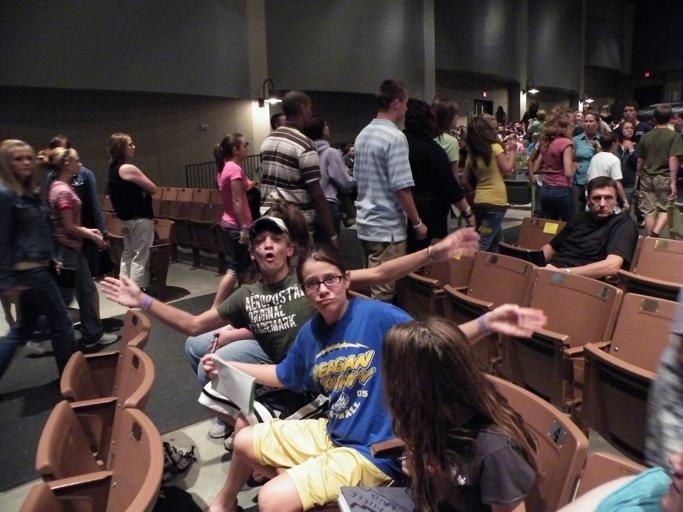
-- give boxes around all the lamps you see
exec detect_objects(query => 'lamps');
[259,79,282,107]
[580,92,594,104]
[522,78,540,95]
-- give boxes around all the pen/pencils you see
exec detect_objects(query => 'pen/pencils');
[210,333,220,353]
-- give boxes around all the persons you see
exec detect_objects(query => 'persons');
[554,445,682,511]
[206,241,547,511]
[258,78,683,321]
[107,131,157,290]
[97,214,480,486]
[382,317,544,512]
[647,290,683,466]
[185,201,313,451]
[213,132,255,308]
[0,130,108,388]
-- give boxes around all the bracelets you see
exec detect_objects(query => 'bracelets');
[141,295,153,310]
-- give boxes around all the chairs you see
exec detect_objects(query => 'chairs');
[21,407,168,512]
[61,307,151,458]
[34,347,158,481]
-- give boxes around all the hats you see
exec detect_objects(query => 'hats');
[248,215,288,239]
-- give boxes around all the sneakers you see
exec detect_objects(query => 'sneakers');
[85,334,118,348]
[208,417,234,450]
[25,340,45,354]
[637,216,645,228]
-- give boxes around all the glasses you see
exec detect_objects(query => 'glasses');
[299,276,344,294]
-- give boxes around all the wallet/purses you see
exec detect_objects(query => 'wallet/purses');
[48,265,77,289]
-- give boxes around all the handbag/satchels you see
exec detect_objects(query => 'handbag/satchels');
[153,486,203,511]
[91,248,110,276]
[163,442,196,482]
